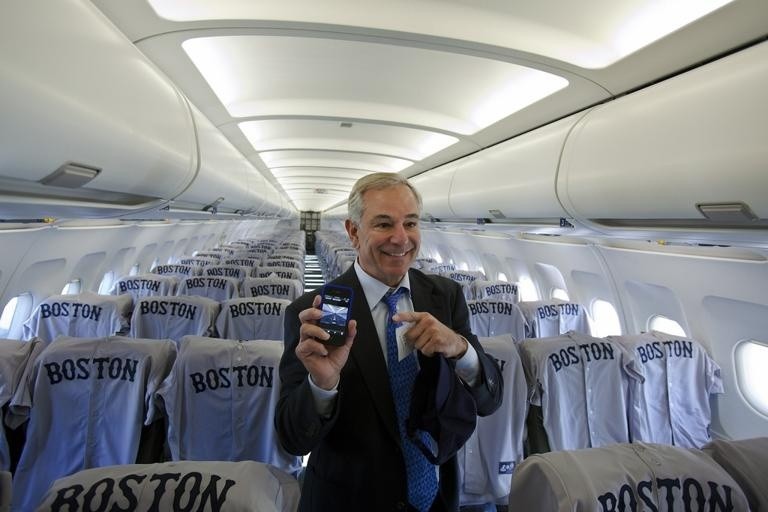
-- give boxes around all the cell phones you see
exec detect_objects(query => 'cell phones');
[313,284,354,346]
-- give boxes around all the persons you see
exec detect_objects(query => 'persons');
[272,171,504,511]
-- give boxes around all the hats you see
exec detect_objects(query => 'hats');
[408,348,478,463]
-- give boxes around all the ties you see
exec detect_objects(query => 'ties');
[382,289,438,512]
